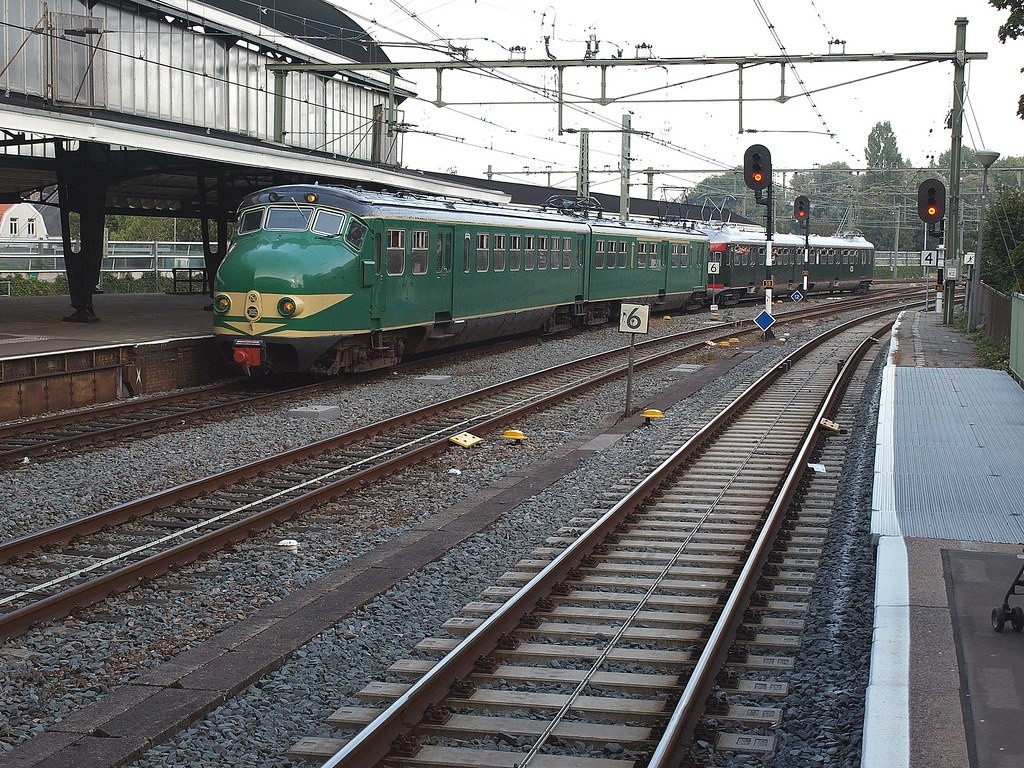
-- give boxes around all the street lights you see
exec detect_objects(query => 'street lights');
[966,148,1003,334]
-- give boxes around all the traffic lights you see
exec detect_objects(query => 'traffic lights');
[926,188,937,216]
[751,153,764,182]
[797,199,805,219]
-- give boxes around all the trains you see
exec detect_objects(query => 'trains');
[212,183,877,390]
